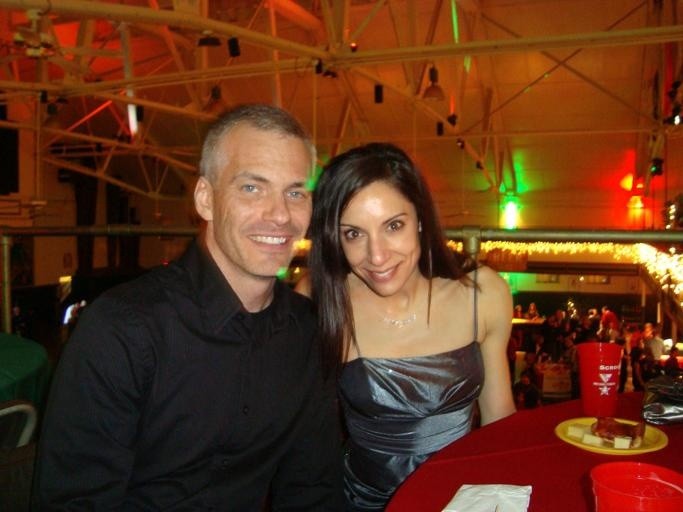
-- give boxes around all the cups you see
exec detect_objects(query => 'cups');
[574,343,624,419]
[590,461,683,512]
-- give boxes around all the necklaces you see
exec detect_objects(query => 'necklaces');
[354,276,421,330]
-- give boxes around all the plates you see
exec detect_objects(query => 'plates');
[555,416,669,455]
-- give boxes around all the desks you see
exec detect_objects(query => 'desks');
[0,330,51,404]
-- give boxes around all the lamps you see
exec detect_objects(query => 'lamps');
[375,85,385,103]
[196,34,241,58]
[41,89,68,115]
[661,101,683,125]
[648,157,664,177]
[437,122,444,136]
[422,60,447,102]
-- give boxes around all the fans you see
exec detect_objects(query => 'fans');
[0,9,129,80]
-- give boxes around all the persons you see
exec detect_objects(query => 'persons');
[288,141,519,511]
[504,296,682,410]
[33,101,356,511]
